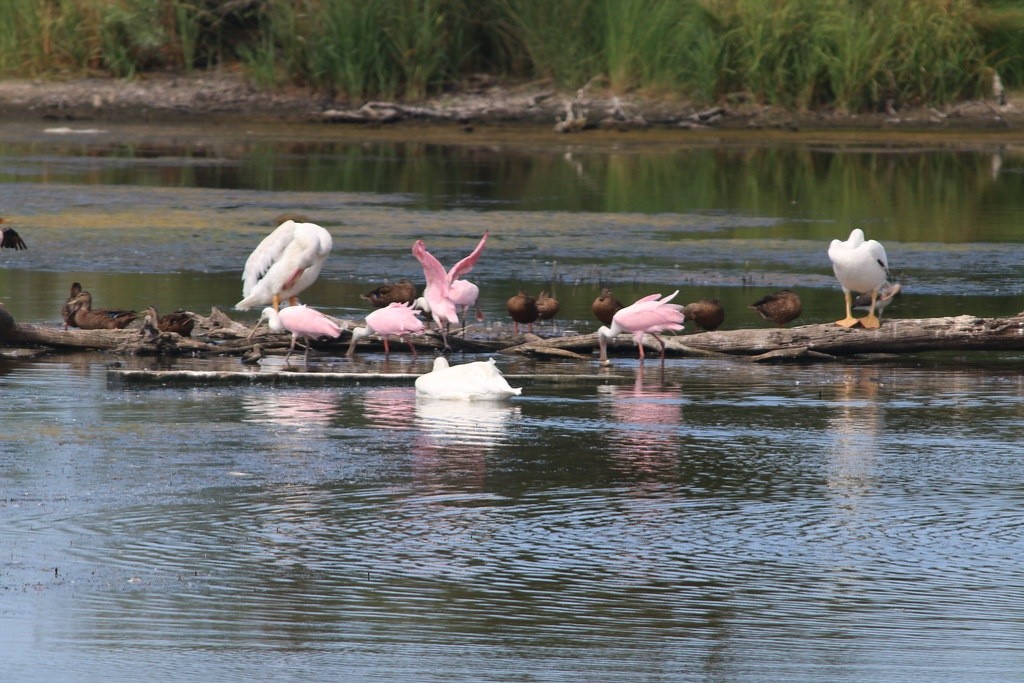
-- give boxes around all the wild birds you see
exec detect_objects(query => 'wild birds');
[60,281,194,366]
[0,227,28,251]
[745,289,801,325]
[827,228,902,331]
[232,219,725,403]
